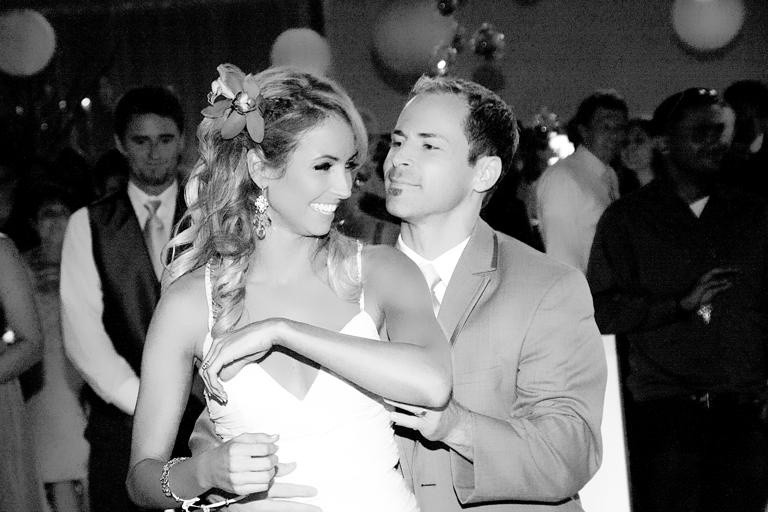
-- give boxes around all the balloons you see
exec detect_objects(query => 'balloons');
[0,9,56,76]
[271,29,333,77]
[368,1,458,80]
[670,1,746,52]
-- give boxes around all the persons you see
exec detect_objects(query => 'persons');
[1,67,768,512]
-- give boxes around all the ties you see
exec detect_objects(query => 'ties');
[421,265,441,316]
[143,200,168,283]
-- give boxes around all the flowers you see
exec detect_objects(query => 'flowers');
[200,62,264,143]
